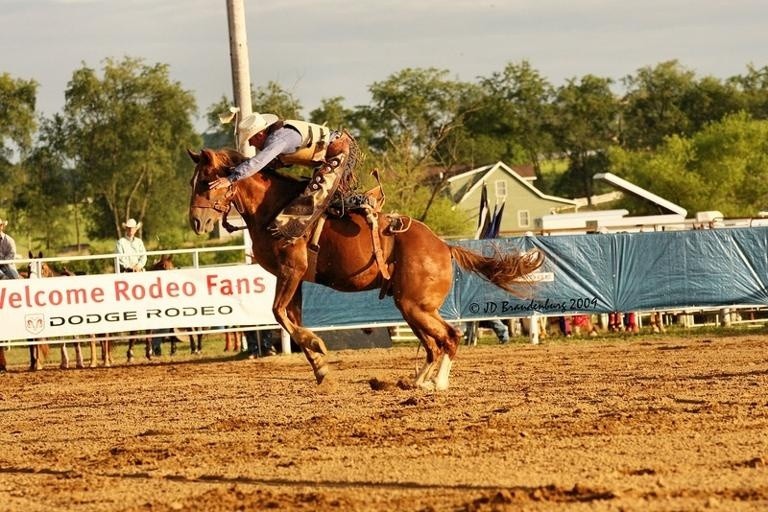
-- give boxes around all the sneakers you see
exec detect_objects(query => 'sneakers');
[244,348,278,356]
[362,196,375,226]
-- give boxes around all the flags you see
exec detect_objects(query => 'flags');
[474,181,506,238]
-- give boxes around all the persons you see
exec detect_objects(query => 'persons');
[209,109,360,243]
[240,327,276,358]
[0,219,20,279]
[114,218,148,273]
[456,311,664,345]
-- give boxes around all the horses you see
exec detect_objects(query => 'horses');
[101,253,177,368]
[586,221,705,338]
[0,262,35,372]
[26,251,99,371]
[184,146,545,393]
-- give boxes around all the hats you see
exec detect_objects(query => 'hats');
[238,112,279,152]
[121,218,143,230]
